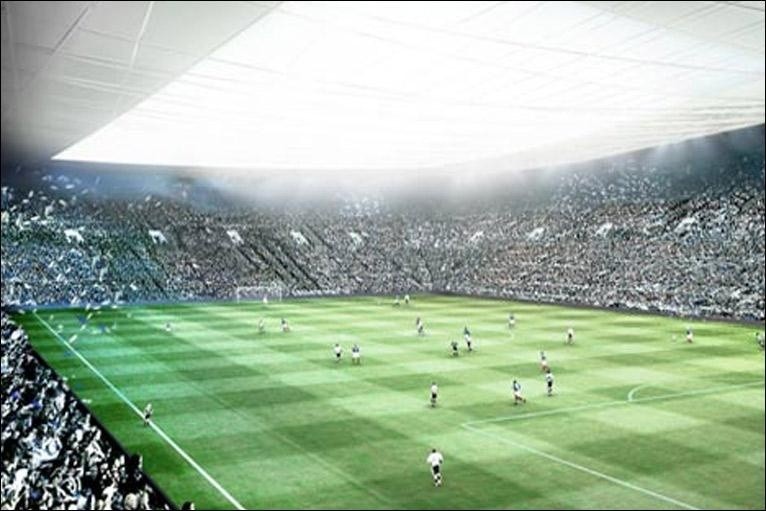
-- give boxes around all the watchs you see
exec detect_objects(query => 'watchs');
[511,378,526,405]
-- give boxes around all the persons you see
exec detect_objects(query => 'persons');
[685,324,693,343]
[508,311,517,337]
[544,368,554,394]
[425,447,445,486]
[333,343,342,362]
[565,327,576,344]
[451,339,458,356]
[415,318,427,333]
[538,351,550,371]
[350,344,361,364]
[0,153,765,326]
[755,327,765,348]
[280,320,289,331]
[255,316,267,334]
[464,324,472,352]
[429,381,439,405]
[0,327,201,510]
